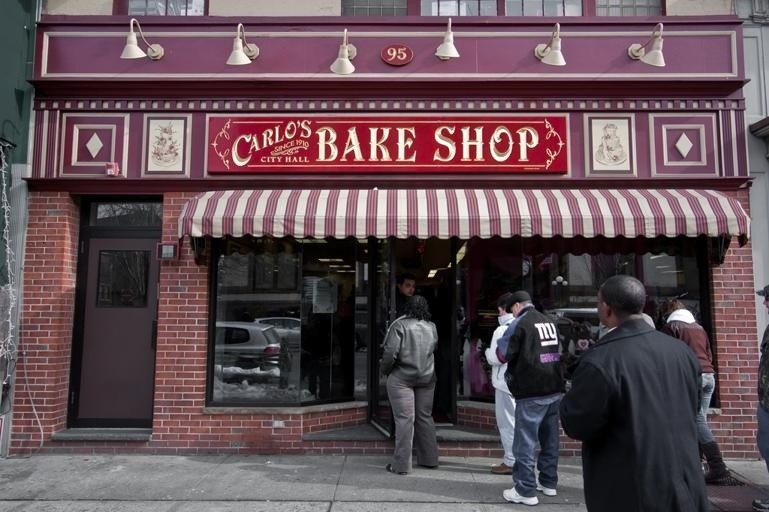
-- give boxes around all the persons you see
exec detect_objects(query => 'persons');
[457,307,465,333]
[395,272,418,319]
[661,298,730,483]
[478,287,517,474]
[645,300,662,330]
[559,274,709,512]
[383,294,440,475]
[752,285,769,511]
[497,290,565,505]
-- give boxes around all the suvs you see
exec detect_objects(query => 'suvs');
[215,319,290,369]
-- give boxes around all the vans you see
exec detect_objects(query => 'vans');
[550,308,603,335]
[256,316,300,347]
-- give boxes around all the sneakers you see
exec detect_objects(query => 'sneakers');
[419,465,439,469]
[503,488,539,506]
[535,479,556,496]
[385,464,407,476]
[491,463,513,476]
[752,498,769,510]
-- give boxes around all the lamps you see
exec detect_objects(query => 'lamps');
[329,29,358,75]
[226,24,259,67]
[158,241,181,263]
[628,23,668,68]
[435,18,461,61]
[534,23,568,67]
[118,20,164,60]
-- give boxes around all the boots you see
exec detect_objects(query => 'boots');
[700,442,729,480]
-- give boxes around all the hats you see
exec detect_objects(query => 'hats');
[505,291,531,312]
[756,286,769,301]
